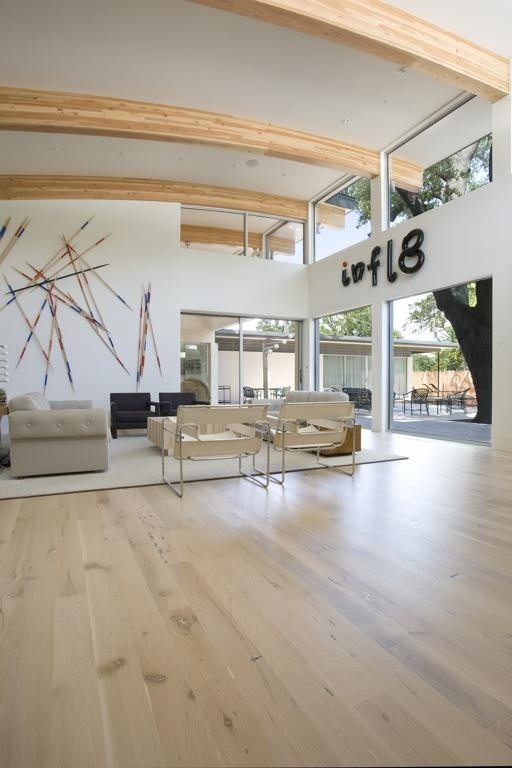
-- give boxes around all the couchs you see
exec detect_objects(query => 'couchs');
[247,389,351,451]
[7,392,112,480]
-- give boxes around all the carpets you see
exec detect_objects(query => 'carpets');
[0,433,408,503]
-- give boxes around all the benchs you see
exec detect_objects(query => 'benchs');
[162,400,359,500]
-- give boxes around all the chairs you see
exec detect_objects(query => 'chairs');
[401,385,472,415]
[242,384,291,403]
[110,390,211,441]
